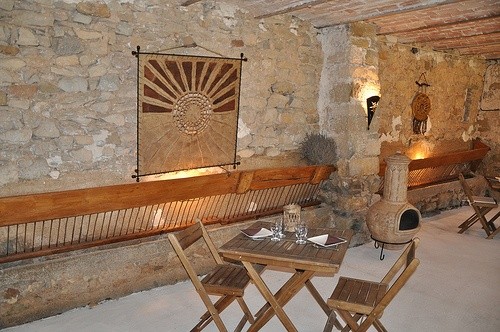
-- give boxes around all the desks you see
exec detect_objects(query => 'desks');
[218,221,355,332]
[481,175,500,239]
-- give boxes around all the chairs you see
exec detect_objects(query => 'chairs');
[326,237,420,332]
[168,218,256,332]
[458,169,498,239]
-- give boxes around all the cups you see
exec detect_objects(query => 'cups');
[294,223,307,245]
[271,215,283,241]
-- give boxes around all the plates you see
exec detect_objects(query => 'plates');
[307,234,347,248]
[239,227,273,238]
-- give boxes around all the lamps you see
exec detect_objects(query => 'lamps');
[367,96,380,130]
